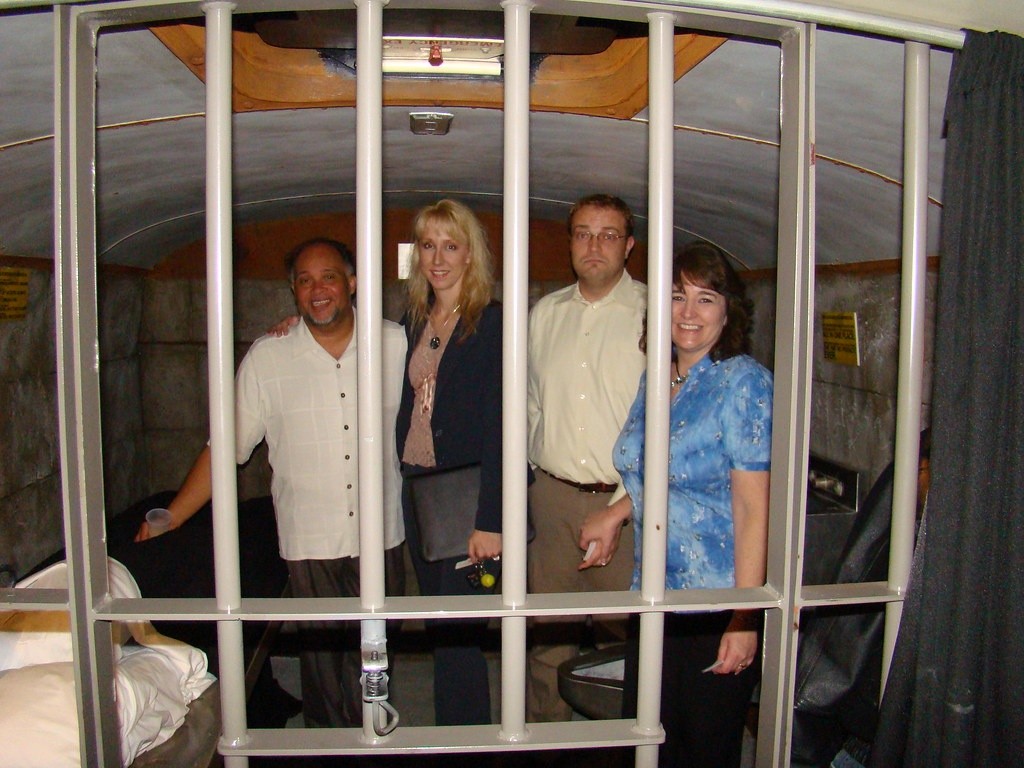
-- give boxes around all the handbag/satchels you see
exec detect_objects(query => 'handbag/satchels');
[409,463,538,565]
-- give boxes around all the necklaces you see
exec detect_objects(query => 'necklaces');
[428,305,460,349]
[676,361,685,378]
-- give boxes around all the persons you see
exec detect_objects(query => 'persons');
[524,196,647,768]
[579,244,774,768]
[265,199,502,728]
[134,238,414,768]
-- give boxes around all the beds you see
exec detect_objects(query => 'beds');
[0,490,290,768]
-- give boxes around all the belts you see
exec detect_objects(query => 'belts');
[540,467,618,493]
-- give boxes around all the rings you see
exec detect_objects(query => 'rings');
[739,663,746,668]
[493,555,500,561]
[601,561,607,565]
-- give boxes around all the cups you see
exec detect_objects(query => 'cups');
[455,557,481,590]
[146,509,172,537]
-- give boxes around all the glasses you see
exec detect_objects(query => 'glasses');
[569,230,628,244]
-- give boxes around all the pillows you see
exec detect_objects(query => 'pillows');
[0,646,219,768]
[0,528,184,670]
